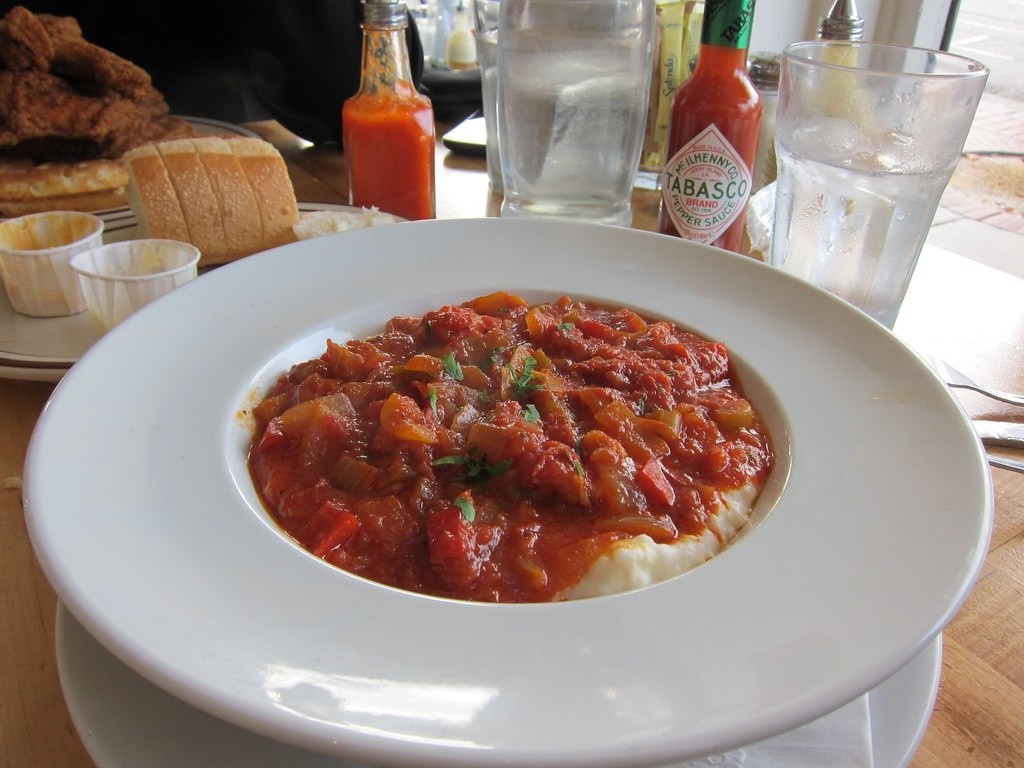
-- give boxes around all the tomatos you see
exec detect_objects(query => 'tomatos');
[249,291,775,604]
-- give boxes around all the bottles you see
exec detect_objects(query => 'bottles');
[655,0,764,260]
[807,3,880,210]
[339,0,437,220]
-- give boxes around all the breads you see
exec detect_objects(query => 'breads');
[124,137,301,269]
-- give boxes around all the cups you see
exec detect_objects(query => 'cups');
[472,0,654,231]
[767,41,993,341]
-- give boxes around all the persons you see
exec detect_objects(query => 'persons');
[0,0,424,147]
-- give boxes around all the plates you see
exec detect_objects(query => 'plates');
[1,114,998,766]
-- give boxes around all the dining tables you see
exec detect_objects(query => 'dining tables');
[3,106,1024,766]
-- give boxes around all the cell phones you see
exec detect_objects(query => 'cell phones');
[441,106,487,156]
